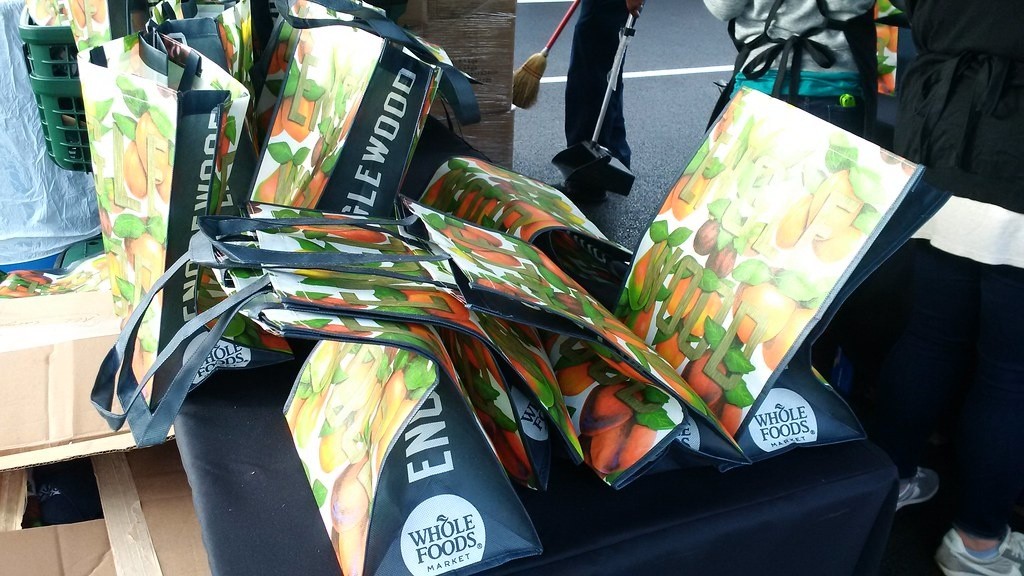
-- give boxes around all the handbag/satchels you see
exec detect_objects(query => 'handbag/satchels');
[60,0,953,576]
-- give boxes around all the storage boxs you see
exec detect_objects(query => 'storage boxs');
[0,336,213,576]
[439,110,514,170]
[395,0,516,116]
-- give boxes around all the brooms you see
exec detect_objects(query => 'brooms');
[512,1,583,110]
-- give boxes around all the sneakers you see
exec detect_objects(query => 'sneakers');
[934,525,1024,576]
[896,466,940,512]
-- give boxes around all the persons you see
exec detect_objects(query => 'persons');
[702,0,881,141]
[547,0,645,206]
[873,0,1024,576]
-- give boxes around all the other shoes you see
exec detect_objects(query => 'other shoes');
[547,184,606,203]
